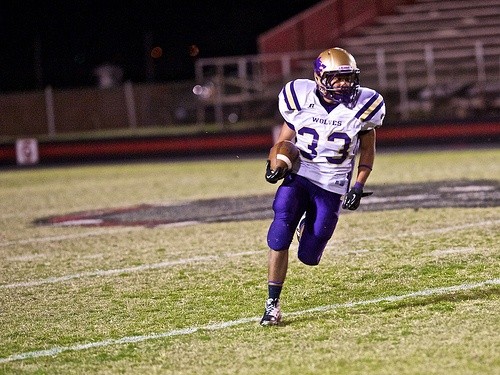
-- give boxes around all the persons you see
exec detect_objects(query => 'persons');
[259,47,386,328]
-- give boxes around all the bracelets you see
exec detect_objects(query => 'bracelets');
[358,164,373,171]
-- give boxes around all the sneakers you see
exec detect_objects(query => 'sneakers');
[260,298,282,327]
[296,211,306,242]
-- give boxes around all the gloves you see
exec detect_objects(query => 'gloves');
[342,187,363,211]
[265,160,293,184]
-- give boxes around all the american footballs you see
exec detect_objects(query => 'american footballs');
[269,139,299,172]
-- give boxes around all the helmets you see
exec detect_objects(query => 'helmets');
[313,47,362,103]
